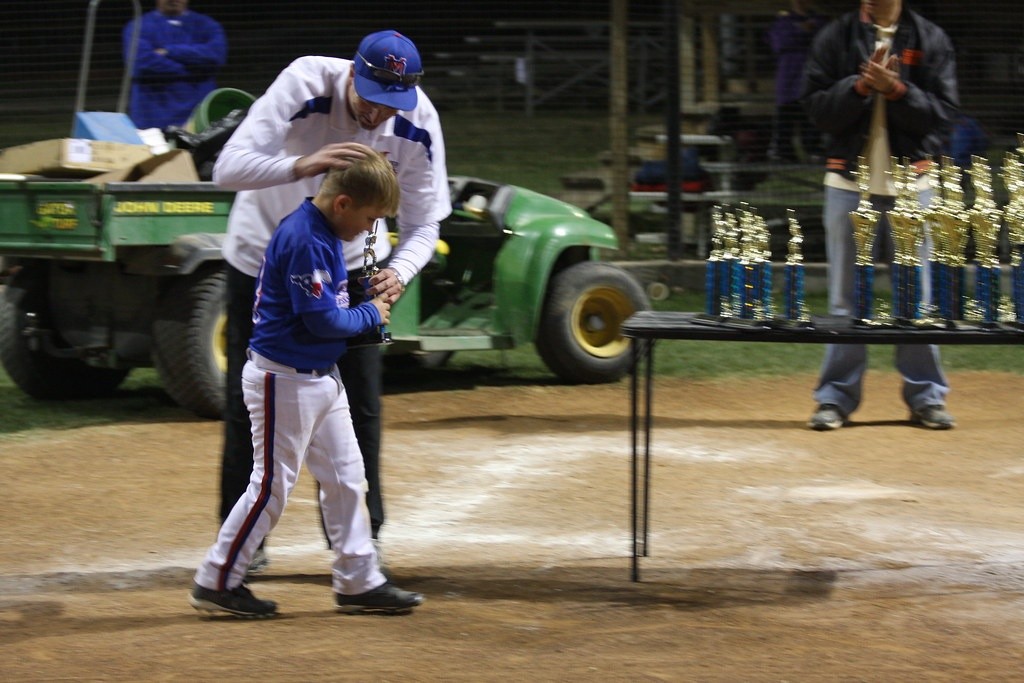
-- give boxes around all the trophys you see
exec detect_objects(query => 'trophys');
[359,232,395,345]
[702,201,811,324]
[847,155,883,327]
[888,156,925,325]
[928,153,1024,327]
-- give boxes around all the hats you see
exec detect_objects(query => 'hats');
[354,31,422,111]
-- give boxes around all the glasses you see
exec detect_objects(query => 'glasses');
[356,50,424,87]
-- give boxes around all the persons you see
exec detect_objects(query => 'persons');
[123,0,225,129]
[190,147,426,615]
[800,0,959,432]
[212,30,454,572]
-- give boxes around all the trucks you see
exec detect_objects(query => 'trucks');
[0,176,656,420]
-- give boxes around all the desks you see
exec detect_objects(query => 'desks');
[617,311,1024,580]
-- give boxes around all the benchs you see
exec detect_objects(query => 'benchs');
[560,98,824,259]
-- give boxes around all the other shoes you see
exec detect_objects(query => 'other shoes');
[246,548,269,574]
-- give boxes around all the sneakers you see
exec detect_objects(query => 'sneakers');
[188,582,277,617]
[909,407,955,428]
[807,404,851,428]
[336,582,422,612]
